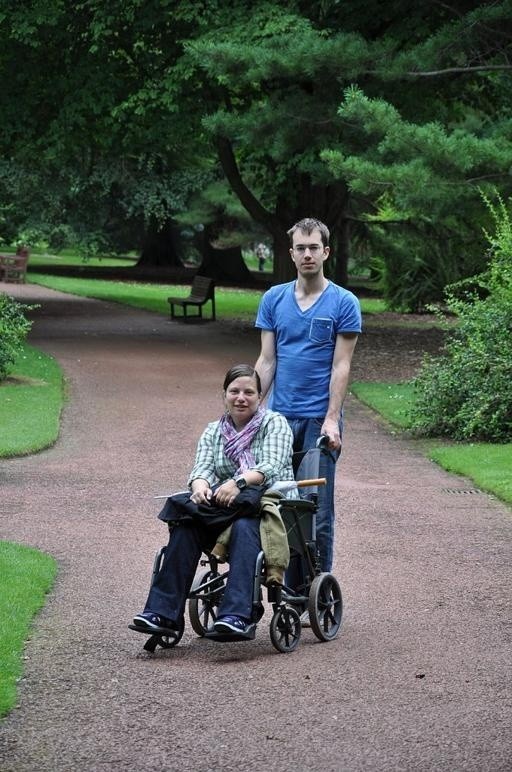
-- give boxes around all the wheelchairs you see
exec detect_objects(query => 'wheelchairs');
[128,435,344,656]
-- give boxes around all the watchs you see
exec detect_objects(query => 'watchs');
[232,475,247,491]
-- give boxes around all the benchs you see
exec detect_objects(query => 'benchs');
[166,276,218,322]
[0,246,31,284]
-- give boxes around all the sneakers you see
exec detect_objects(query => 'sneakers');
[133,612,175,633]
[281,602,302,625]
[214,615,250,632]
[299,606,321,627]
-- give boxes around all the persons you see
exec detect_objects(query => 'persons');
[256,243,266,272]
[133,364,301,635]
[253,217,362,628]
